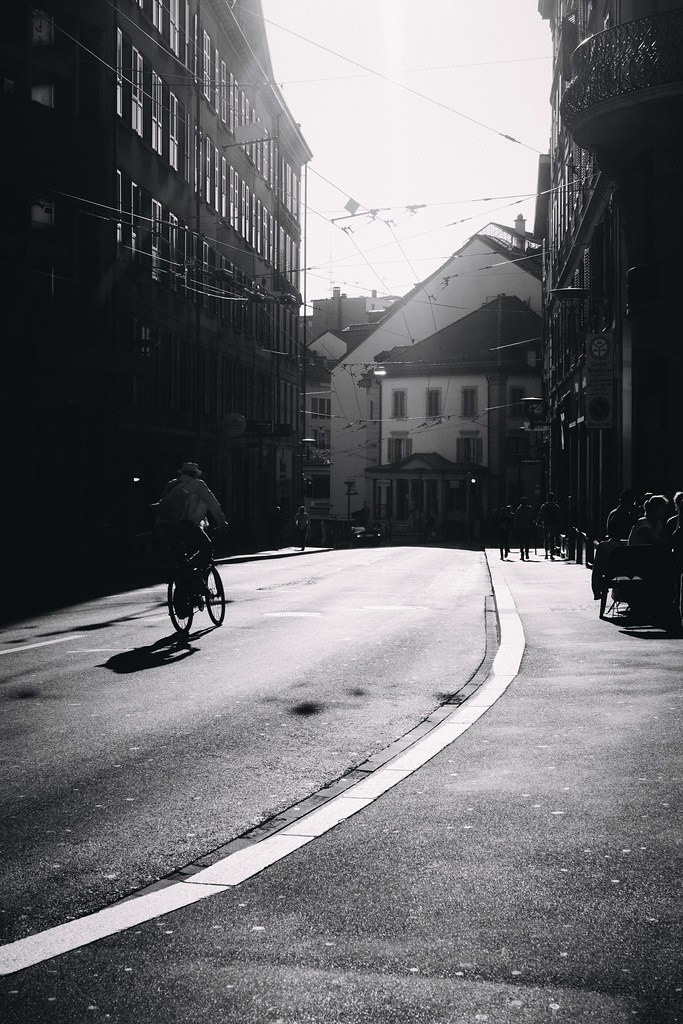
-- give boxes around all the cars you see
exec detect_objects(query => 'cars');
[346,526,382,547]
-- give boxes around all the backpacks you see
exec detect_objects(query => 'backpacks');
[159,490,190,522]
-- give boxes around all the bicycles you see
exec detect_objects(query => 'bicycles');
[167,526,227,634]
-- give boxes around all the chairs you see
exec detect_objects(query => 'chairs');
[590,534,657,626]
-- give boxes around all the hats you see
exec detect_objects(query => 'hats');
[177,462,203,476]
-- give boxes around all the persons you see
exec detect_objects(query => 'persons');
[499,493,561,560]
[269,503,311,550]
[373,516,393,544]
[424,512,434,544]
[161,462,229,596]
[592,488,683,629]
